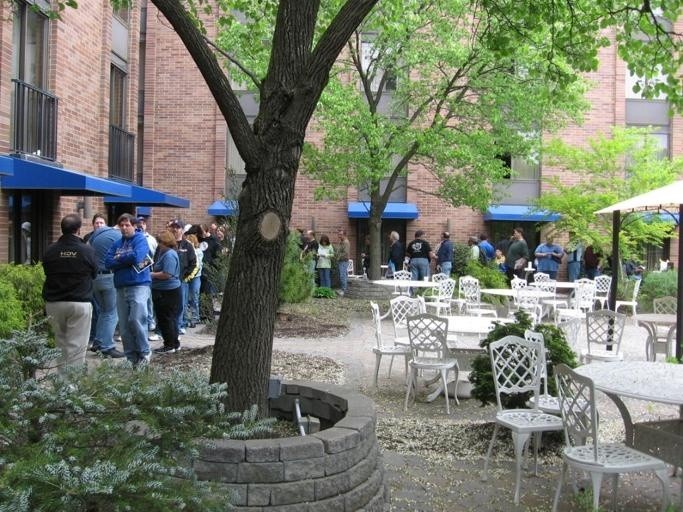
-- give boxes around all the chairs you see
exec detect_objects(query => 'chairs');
[447,275,481,314]
[534,272,549,281]
[558,278,596,326]
[346,260,364,279]
[432,273,451,303]
[536,277,568,328]
[370,301,416,386]
[459,281,498,337]
[393,270,412,297]
[389,294,440,383]
[615,278,641,322]
[525,329,594,491]
[591,275,612,313]
[653,296,677,360]
[405,315,459,414]
[553,363,671,512]
[482,336,580,505]
[511,275,542,322]
[580,309,626,363]
[513,291,539,325]
[424,279,456,314]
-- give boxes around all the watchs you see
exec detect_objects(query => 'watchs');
[543,253,545,257]
[116,254,120,260]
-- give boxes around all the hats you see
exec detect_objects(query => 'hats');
[169,219,185,229]
[138,217,146,220]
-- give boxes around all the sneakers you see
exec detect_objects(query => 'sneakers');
[148,335,163,341]
[155,346,176,354]
[137,351,152,368]
[114,334,121,342]
[179,326,186,335]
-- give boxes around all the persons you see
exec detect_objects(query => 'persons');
[478,233,495,264]
[86,218,138,357]
[201,225,224,294]
[584,245,601,279]
[149,220,200,340]
[182,224,192,241]
[336,230,350,296]
[151,230,183,354]
[433,233,444,260]
[20,221,32,266]
[405,230,433,281]
[185,225,208,328]
[495,249,505,267]
[42,214,97,374]
[303,230,318,263]
[563,232,582,282]
[468,236,479,260]
[137,217,159,330]
[105,214,152,372]
[388,231,401,280]
[535,234,564,280]
[626,258,643,280]
[166,220,174,231]
[83,213,107,348]
[316,234,335,288]
[505,228,529,287]
[216,227,232,292]
[210,224,217,235]
[437,232,452,277]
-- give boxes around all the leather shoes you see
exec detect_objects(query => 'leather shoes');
[103,347,124,357]
[90,344,101,352]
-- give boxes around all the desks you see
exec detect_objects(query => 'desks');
[632,314,678,363]
[481,289,554,326]
[380,265,389,278]
[525,268,536,282]
[573,364,683,475]
[529,283,584,320]
[372,279,440,320]
